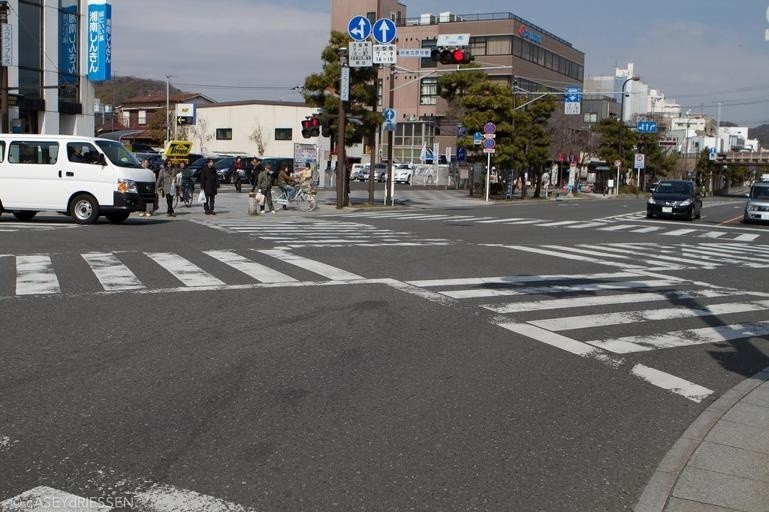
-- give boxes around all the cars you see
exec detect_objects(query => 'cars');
[743,180,769,223]
[356,162,417,185]
[646,179,703,221]
[184,156,293,186]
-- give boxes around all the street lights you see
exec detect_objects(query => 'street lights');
[618,75,641,155]
[683,114,701,180]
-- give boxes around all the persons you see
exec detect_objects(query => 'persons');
[200,159,219,215]
[174,159,193,202]
[248,156,266,195]
[231,155,245,193]
[276,165,299,210]
[139,156,159,217]
[156,159,178,215]
[295,161,313,194]
[71,143,97,163]
[256,164,276,215]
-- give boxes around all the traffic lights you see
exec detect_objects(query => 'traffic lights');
[301,117,320,139]
[431,48,475,65]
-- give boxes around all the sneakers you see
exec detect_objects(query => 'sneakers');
[205,210,217,216]
[167,208,176,218]
[260,210,266,215]
[138,212,152,217]
[283,205,288,210]
[288,197,295,202]
[270,209,276,214]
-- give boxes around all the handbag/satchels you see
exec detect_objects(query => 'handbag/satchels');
[255,189,265,206]
[231,175,235,183]
[170,179,180,197]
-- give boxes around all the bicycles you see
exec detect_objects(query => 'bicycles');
[265,176,316,212]
[172,172,194,210]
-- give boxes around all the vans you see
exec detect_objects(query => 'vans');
[0,132,159,225]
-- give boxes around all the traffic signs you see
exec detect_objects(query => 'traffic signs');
[483,138,496,149]
[484,122,497,134]
